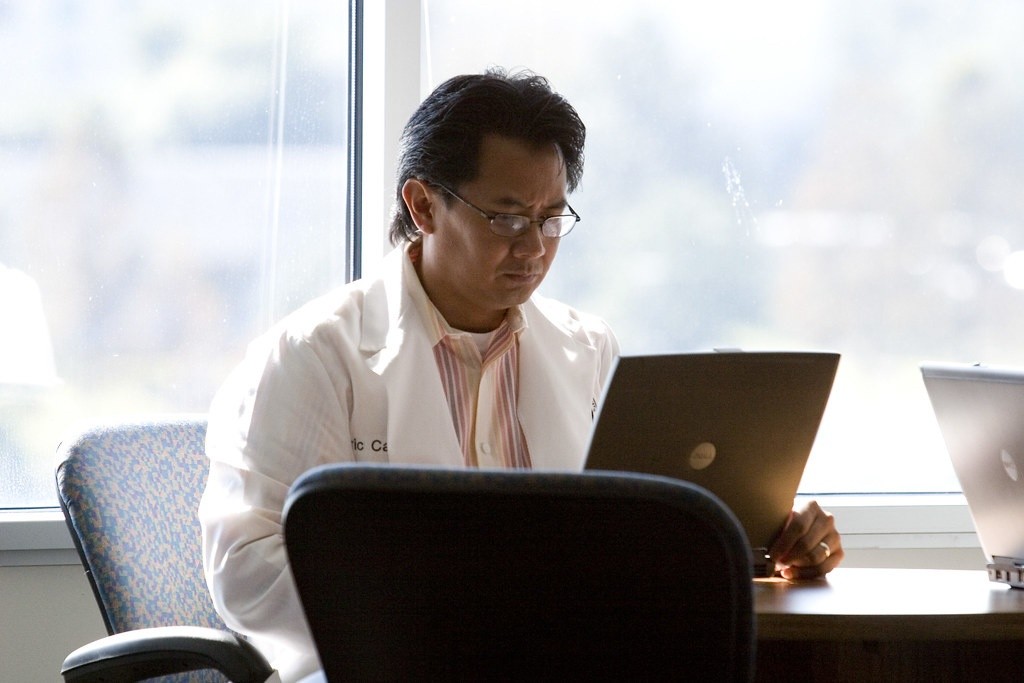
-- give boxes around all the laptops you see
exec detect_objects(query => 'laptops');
[921,363,1024,588]
[580,351,841,579]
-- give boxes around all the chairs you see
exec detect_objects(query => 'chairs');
[56,423,278,683]
[280,463,755,683]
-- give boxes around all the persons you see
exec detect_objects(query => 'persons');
[200,73,843,683]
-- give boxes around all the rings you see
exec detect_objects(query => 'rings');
[820,542,831,558]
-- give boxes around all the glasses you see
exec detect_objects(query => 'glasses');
[428,182,580,237]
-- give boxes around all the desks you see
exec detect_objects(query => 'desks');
[753,566,1024,683]
